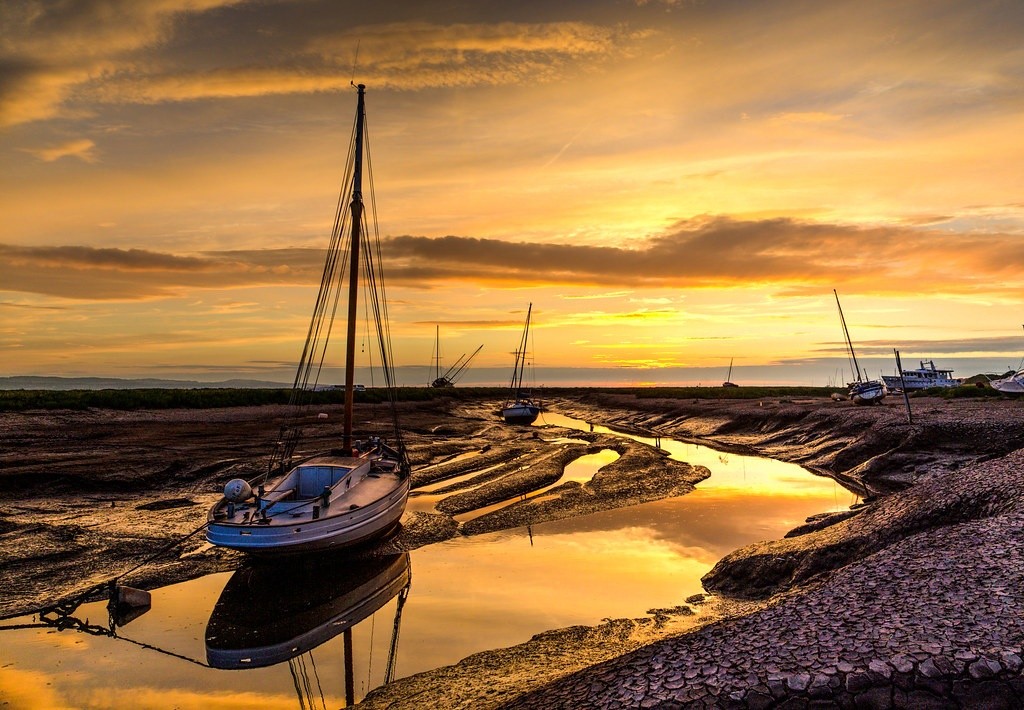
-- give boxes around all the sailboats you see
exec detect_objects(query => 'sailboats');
[427,325,483,387]
[832,288,885,406]
[499,303,539,418]
[207,84,411,560]
[722,358,739,388]
[205,523,412,710]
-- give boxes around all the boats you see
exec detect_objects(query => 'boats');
[881,359,960,393]
[984,358,1024,400]
